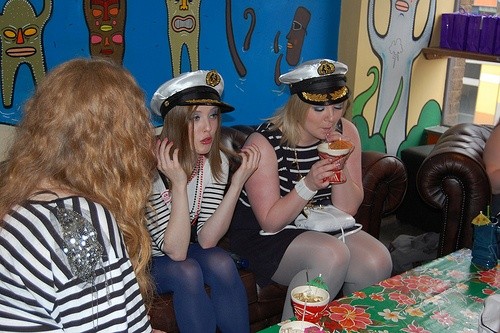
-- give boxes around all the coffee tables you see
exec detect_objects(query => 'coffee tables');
[260,249,500,333]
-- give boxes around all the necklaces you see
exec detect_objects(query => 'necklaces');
[167,155,203,226]
[294,147,303,179]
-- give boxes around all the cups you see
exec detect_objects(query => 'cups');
[290,285,330,326]
[317,143,355,185]
[471,217,500,269]
[278,321,322,333]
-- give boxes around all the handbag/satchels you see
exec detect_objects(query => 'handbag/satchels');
[388,232,440,275]
[294,205,355,232]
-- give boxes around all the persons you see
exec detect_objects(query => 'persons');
[144,70,261,333]
[233,59,393,323]
[483,117,500,195]
[0,57,167,333]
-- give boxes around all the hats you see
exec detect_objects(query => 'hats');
[279,58,349,106]
[150,69,235,123]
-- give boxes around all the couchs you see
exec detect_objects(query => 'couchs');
[416,123,500,260]
[146,127,409,333]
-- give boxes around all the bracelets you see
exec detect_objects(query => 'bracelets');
[295,177,317,200]
